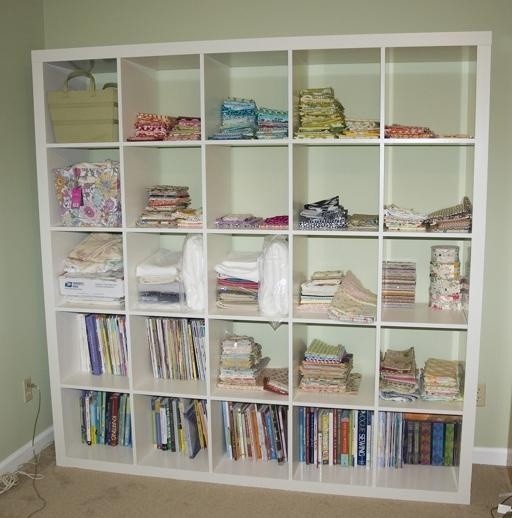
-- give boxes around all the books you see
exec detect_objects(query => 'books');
[74,313,129,377]
[221,402,288,465]
[151,397,208,461]
[297,405,373,471]
[378,412,464,471]
[79,392,132,448]
[145,317,207,381]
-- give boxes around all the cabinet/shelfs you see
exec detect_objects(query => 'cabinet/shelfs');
[31,30,492,507]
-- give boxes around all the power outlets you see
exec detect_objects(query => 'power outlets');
[23,377,34,403]
[477,385,486,406]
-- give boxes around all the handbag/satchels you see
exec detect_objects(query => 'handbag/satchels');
[47,71,118,144]
